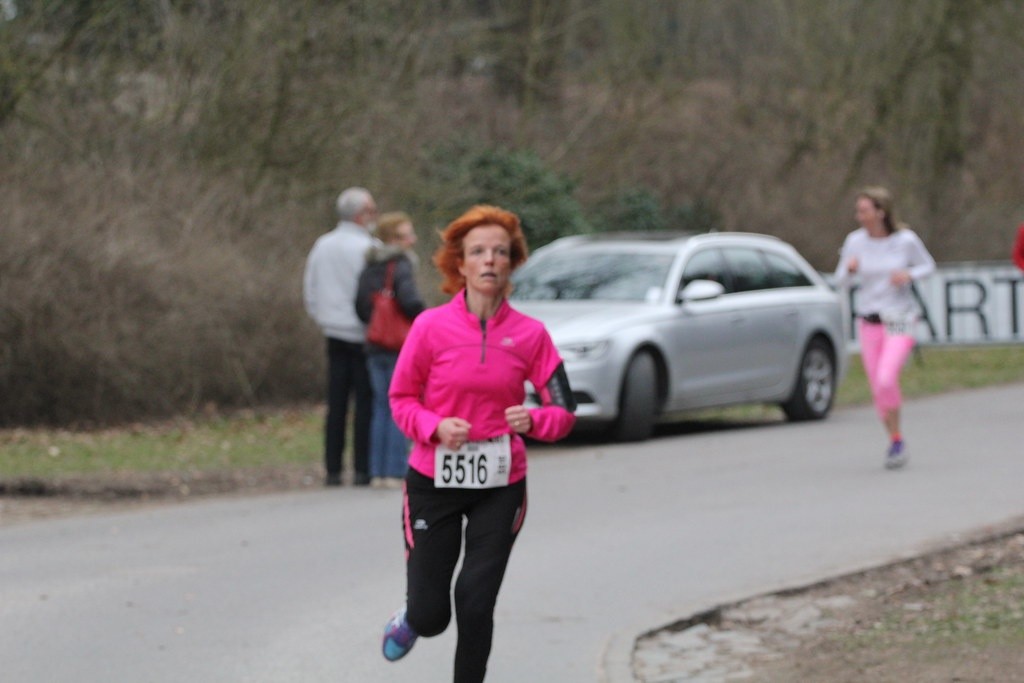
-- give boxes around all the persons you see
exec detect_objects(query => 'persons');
[300,185,385,487]
[355,212,428,491]
[384,205,578,683]
[830,187,938,468]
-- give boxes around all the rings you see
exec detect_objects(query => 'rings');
[515,420,520,426]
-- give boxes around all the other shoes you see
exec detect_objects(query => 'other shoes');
[369,476,404,488]
[327,472,342,487]
[884,439,906,467]
[354,472,372,485]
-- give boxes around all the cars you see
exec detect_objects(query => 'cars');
[502,231,848,445]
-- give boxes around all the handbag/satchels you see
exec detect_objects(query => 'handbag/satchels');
[366,259,414,351]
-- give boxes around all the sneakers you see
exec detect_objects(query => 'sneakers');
[382,608,419,662]
